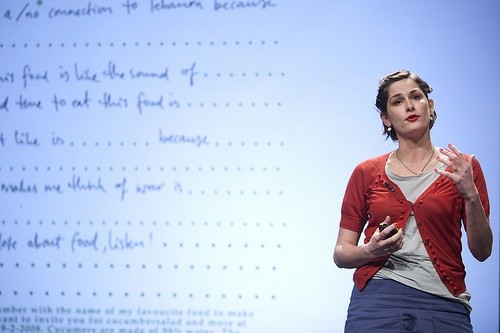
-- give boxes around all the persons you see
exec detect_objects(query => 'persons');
[333,70,494,333]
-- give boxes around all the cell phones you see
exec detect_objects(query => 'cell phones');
[379,223,399,240]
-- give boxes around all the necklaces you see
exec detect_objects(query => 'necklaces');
[396,147,435,176]
